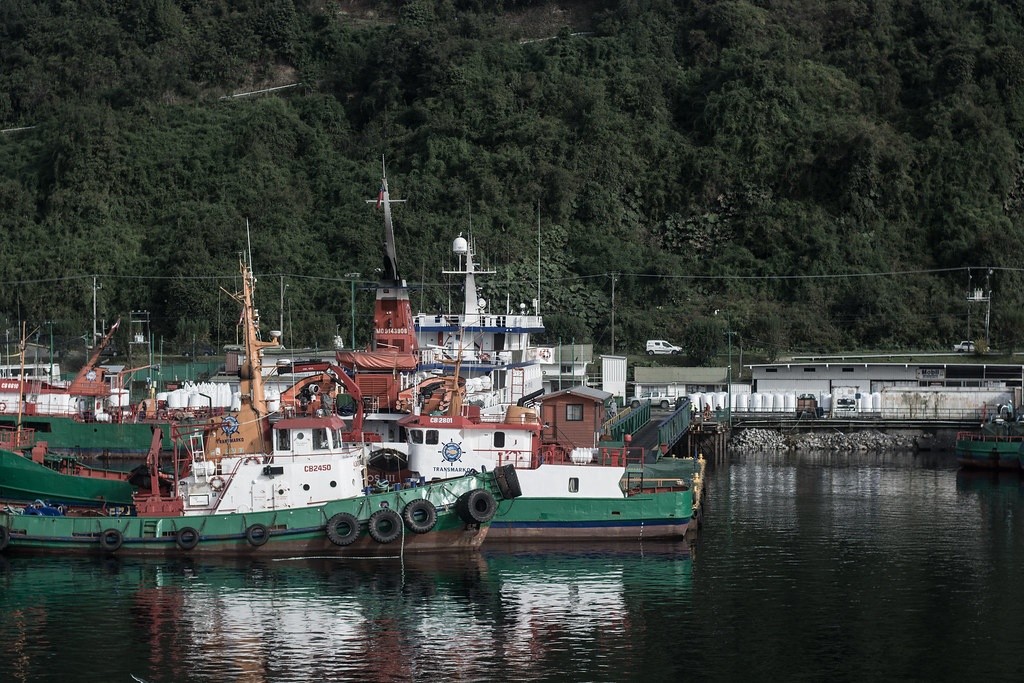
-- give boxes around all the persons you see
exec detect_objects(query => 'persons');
[690,401,722,422]
[164,401,169,410]
[996,400,1013,422]
[142,401,147,412]
[610,399,617,417]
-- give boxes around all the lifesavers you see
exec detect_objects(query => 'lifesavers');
[0,524,10,549]
[367,508,402,543]
[326,512,361,545]
[403,497,438,534]
[176,526,199,549]
[459,488,497,524]
[100,527,123,552]
[493,464,522,499]
[247,522,269,546]
[210,476,226,492]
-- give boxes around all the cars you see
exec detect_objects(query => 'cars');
[953,340,990,353]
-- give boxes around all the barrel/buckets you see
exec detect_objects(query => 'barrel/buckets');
[569,449,593,466]
[25,508,61,516]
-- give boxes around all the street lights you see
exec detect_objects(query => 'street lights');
[714,309,732,427]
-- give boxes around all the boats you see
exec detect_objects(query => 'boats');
[954,398,1024,471]
[0,152,710,561]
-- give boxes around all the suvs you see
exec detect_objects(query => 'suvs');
[646,339,682,356]
